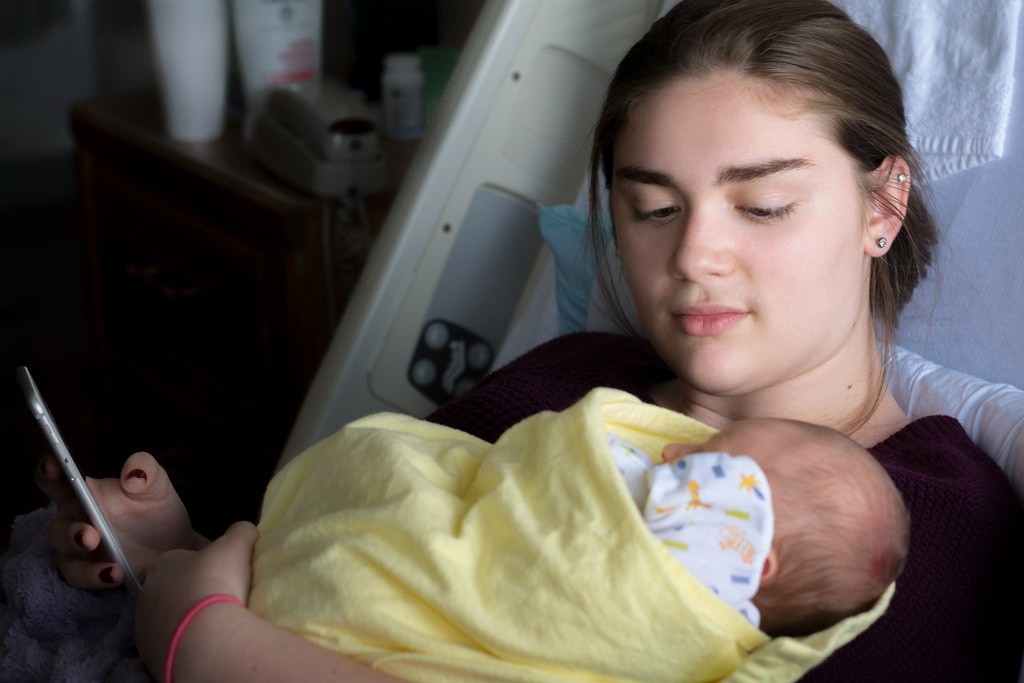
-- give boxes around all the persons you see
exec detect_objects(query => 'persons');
[246,386,909,683]
[34,0,1024,683]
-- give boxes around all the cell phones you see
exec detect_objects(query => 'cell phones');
[15,364,143,598]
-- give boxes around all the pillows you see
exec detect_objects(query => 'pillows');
[534,204,1023,490]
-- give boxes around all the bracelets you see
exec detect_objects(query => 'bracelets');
[162,595,244,683]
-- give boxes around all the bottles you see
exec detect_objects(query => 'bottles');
[381,54,424,141]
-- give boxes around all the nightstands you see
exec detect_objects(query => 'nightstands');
[67,91,430,541]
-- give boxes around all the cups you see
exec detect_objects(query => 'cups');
[145,0,229,143]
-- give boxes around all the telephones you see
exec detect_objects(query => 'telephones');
[249,73,392,197]
